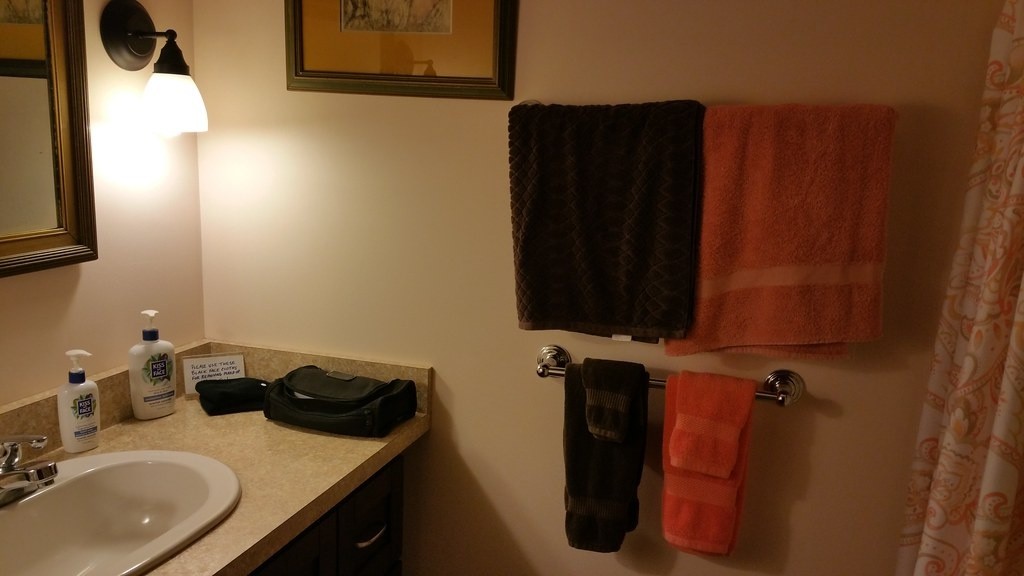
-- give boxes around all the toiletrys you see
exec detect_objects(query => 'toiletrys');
[127,309,179,423]
[54,347,104,454]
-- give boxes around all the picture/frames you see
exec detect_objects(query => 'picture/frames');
[284,0,520,101]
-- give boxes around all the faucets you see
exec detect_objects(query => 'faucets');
[1,431,59,506]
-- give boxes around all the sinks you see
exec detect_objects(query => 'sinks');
[0,447,243,576]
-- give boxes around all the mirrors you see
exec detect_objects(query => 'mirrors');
[0,0,98,278]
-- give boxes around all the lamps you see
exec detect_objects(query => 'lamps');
[100,0,208,137]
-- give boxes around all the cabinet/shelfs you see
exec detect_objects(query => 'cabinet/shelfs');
[252,454,403,576]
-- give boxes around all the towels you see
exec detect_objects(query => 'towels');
[561,355,650,557]
[656,370,763,563]
[507,101,905,367]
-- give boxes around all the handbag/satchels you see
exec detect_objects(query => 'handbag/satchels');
[262,364,418,439]
